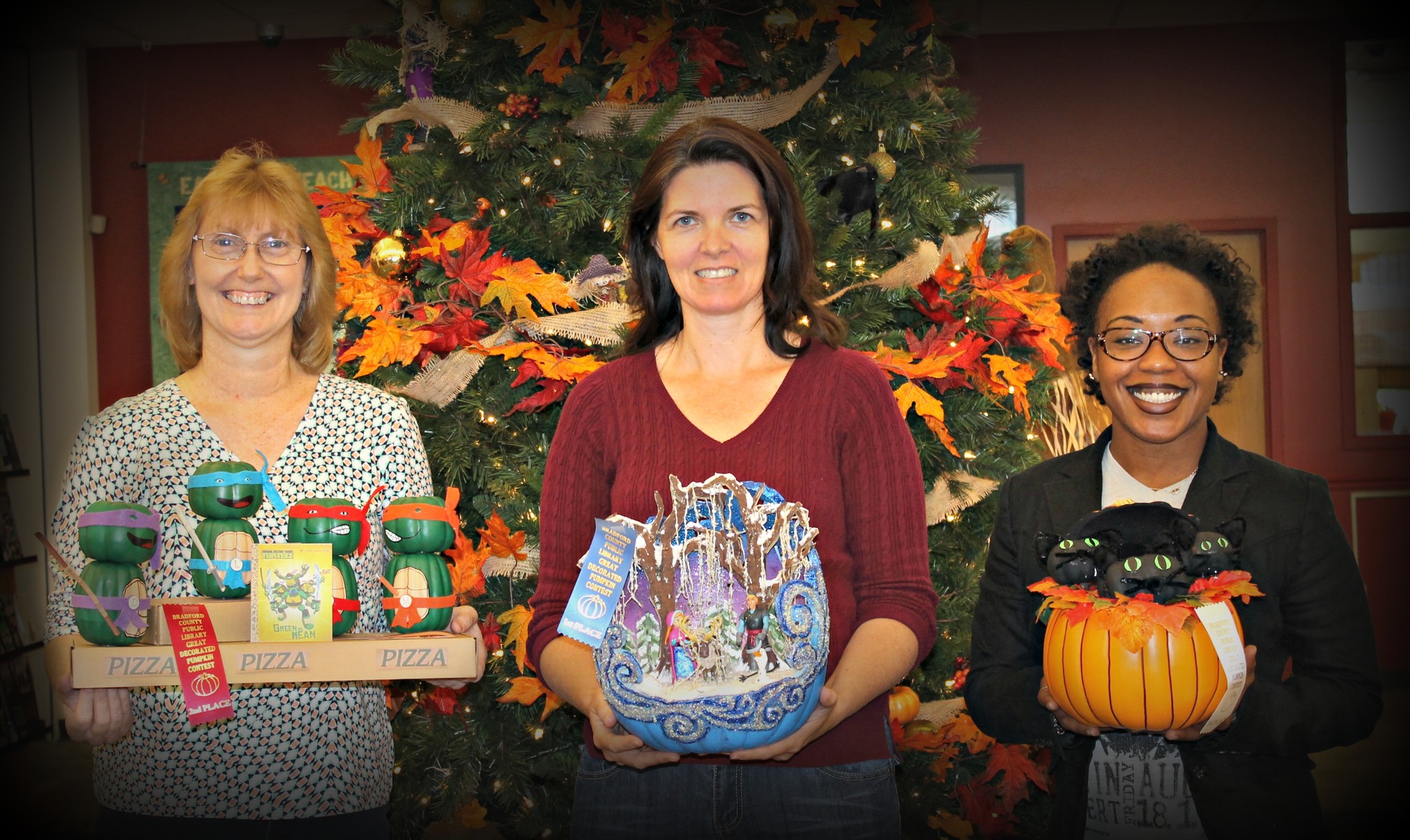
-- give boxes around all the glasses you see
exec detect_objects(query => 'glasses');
[1092,327,1224,362]
[193,232,309,266]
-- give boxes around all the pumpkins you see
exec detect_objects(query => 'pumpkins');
[70,461,456,646]
[1026,500,1266,730]
[889,685,921,726]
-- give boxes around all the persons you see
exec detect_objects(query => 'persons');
[960,216,1388,840]
[43,141,436,840]
[523,115,938,840]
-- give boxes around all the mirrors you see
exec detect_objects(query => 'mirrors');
[959,162,1024,237]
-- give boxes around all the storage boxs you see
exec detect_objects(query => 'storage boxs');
[137,597,251,646]
[67,631,479,690]
